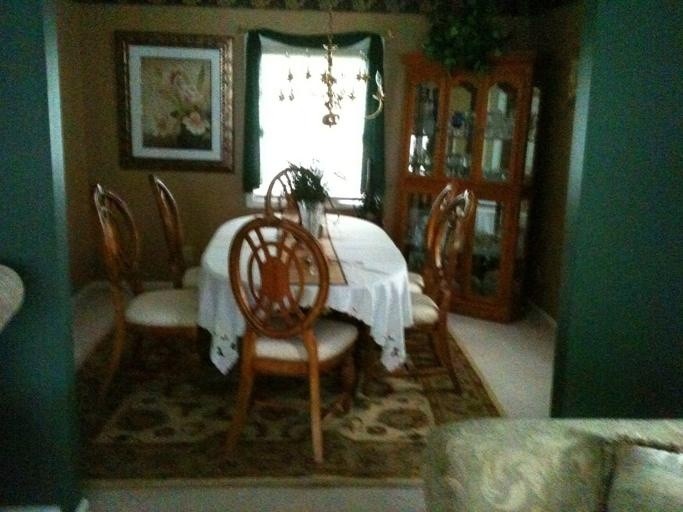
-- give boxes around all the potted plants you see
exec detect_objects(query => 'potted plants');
[288,162,330,241]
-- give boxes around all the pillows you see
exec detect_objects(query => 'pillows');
[602,432,682,512]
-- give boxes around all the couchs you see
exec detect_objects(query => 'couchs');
[422,418,681,511]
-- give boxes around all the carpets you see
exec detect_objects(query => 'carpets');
[73,299,507,489]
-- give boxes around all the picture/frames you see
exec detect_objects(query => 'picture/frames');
[113,28,237,174]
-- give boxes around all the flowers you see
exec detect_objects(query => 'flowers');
[148,63,212,139]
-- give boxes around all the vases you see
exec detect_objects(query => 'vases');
[170,105,211,150]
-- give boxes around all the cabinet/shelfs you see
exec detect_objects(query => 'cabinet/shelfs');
[389,51,551,325]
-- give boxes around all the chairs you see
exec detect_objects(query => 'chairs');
[265,167,325,214]
[223,216,359,463]
[91,184,201,400]
[369,189,477,396]
[150,175,203,287]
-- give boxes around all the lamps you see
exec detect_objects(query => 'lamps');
[277,0,397,127]
